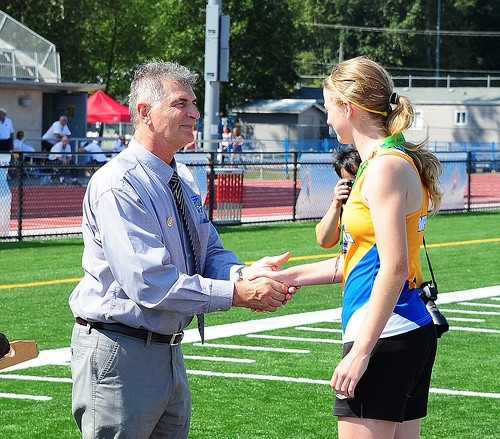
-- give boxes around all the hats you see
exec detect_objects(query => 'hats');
[0,108,6,114]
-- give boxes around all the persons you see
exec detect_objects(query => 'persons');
[0,108,128,185]
[183,124,248,170]
[68,65,301,439]
[248,56,445,439]
[314,146,362,249]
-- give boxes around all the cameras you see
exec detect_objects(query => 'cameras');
[419,280,449,338]
[342,180,354,204]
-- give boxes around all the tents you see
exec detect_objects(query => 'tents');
[86,91,132,137]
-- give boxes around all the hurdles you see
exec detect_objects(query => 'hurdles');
[202,168,244,224]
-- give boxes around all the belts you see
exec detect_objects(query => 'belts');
[75,317,184,345]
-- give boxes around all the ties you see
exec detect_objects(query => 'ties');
[168,171,205,345]
